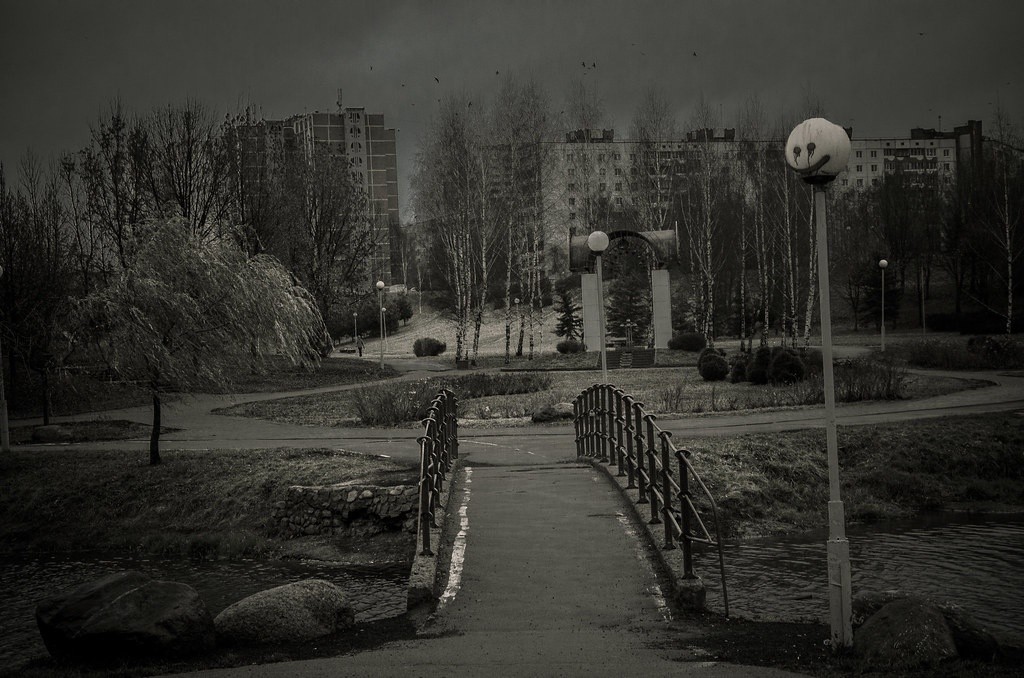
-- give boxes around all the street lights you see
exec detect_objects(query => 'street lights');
[878,260,889,354]
[353,312,358,353]
[587,230,610,457]
[788,117,851,652]
[382,307,388,352]
[376,280,384,371]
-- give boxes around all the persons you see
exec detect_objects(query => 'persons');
[356,335,365,357]
